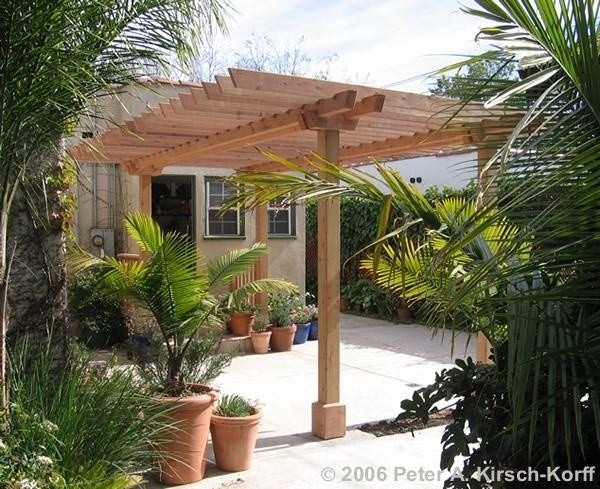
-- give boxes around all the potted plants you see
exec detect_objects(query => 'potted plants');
[339,276,414,321]
[62,209,318,484]
